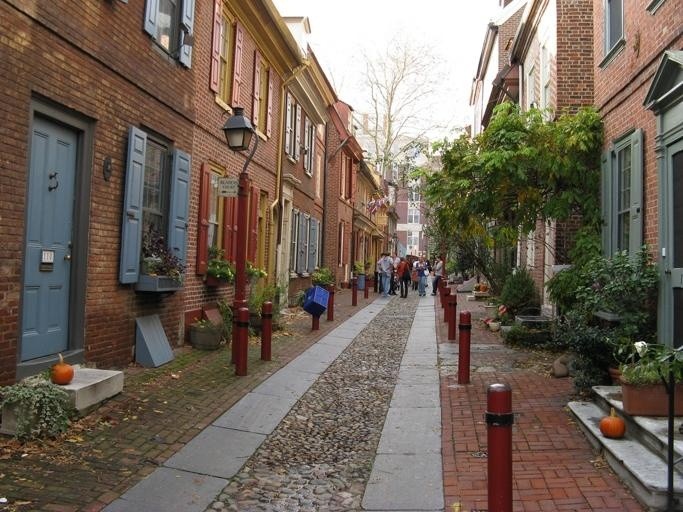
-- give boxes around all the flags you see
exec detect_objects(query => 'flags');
[366,198,388,214]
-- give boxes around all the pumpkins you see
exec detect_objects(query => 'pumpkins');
[50,352,74,385]
[481,285,487,292]
[600,407,625,438]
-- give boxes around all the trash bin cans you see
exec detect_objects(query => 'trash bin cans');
[357,274,366,290]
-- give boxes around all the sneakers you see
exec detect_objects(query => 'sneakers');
[382,290,437,298]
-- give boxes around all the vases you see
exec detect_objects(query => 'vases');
[618,376,683,416]
[490,322,501,333]
[341,281,349,289]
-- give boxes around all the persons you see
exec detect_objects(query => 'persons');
[376,253,443,297]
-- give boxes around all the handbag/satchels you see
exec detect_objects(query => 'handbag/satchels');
[403,271,411,282]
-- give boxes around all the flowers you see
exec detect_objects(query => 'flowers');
[604,330,683,384]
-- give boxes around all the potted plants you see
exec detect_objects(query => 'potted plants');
[311,267,335,291]
[141,222,186,289]
[0,374,69,443]
[189,318,223,350]
[567,242,661,387]
[482,298,497,318]
